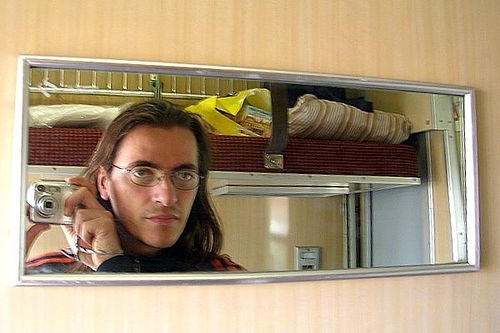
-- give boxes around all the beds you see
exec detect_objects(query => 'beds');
[29,69,421,199]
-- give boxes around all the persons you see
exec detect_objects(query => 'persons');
[24,99,247,273]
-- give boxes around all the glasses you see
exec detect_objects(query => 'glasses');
[110,162,206,190]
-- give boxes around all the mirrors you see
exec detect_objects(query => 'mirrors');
[13,56,482,286]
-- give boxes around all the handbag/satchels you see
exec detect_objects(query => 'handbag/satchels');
[182,87,272,137]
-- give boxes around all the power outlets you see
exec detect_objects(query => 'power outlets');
[296,245,321,271]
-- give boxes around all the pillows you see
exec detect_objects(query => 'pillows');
[289,94,411,144]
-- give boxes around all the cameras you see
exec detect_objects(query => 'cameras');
[26,180,78,224]
